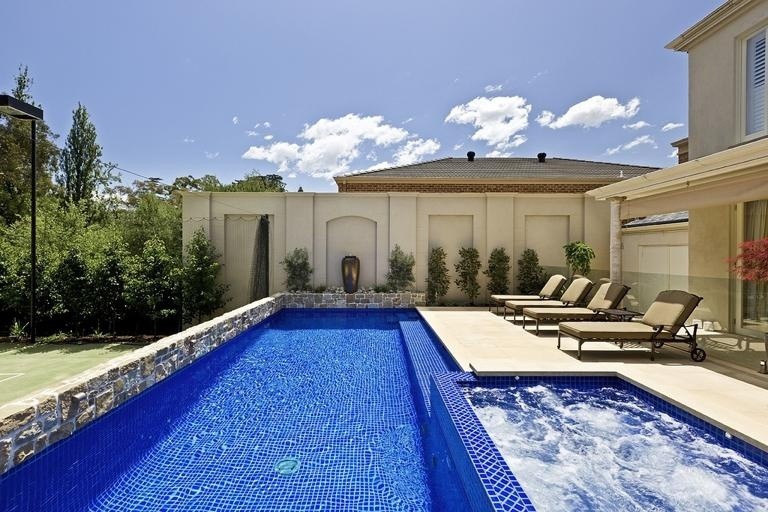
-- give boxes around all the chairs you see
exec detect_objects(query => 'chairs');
[557,290,707,362]
[504,279,587,324]
[523,283,630,335]
[489,274,567,315]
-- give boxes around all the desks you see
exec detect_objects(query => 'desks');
[594,308,644,348]
